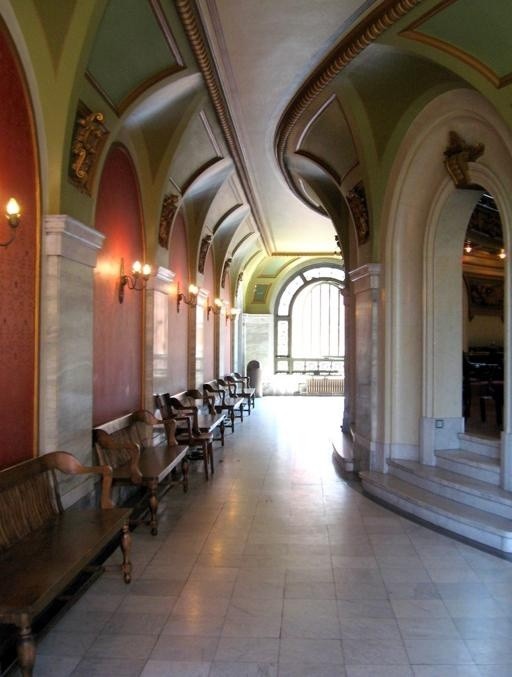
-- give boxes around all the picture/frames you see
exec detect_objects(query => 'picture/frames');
[251,282,272,304]
[462,270,502,323]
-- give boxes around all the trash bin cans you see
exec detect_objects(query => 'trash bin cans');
[247,360,263,397]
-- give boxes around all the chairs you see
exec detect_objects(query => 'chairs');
[461,345,504,434]
[153,393,214,481]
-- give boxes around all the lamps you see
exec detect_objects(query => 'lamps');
[178,282,199,311]
[206,297,223,319]
[2,197,22,249]
[120,258,151,304]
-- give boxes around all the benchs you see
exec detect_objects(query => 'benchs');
[1,452,135,676]
[93,410,190,535]
[225,373,256,416]
[203,378,244,434]
[170,389,226,463]
